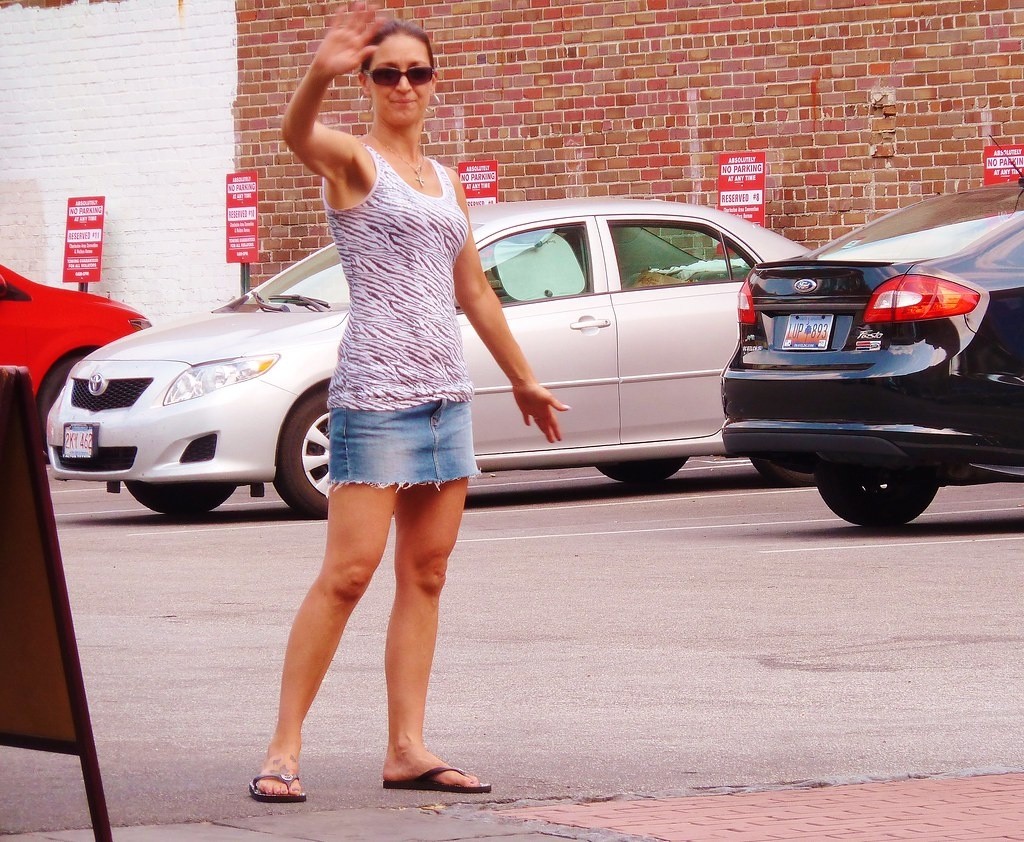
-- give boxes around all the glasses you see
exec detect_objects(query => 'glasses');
[364,66,435,86]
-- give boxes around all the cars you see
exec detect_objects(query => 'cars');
[718,172,1024,530]
[0,265,152,465]
[41,195,818,520]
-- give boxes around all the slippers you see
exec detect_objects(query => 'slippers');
[382,763,492,792]
[248,772,306,803]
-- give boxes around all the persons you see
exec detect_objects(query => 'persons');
[249,1,572,803]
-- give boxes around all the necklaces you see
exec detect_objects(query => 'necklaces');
[369,132,425,187]
